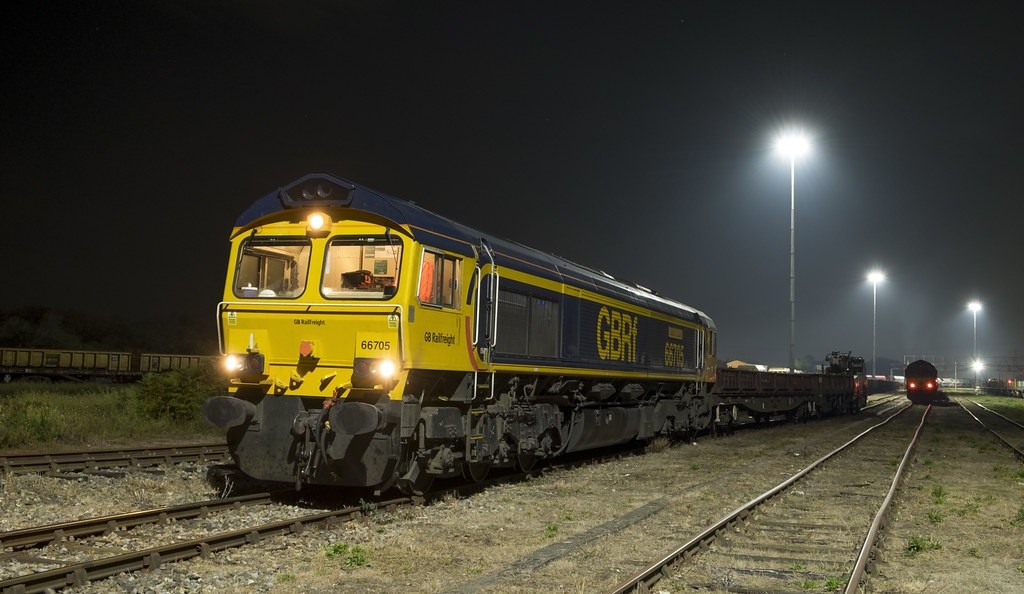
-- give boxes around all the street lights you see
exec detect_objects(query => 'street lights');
[867,270,884,379]
[773,131,810,373]
[968,301,983,386]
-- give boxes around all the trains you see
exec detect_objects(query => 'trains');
[0,348,220,384]
[904,359,938,404]
[206,171,867,499]
